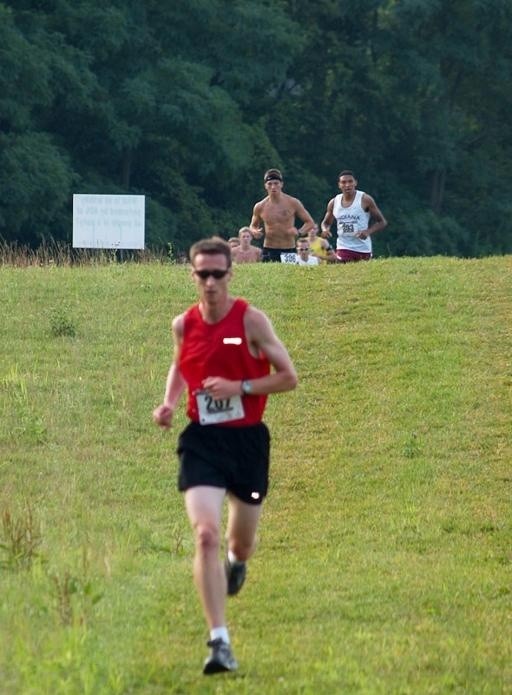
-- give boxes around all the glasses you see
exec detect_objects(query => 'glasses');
[195,270,228,279]
[296,247,309,251]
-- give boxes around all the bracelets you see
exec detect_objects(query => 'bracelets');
[298,228,302,236]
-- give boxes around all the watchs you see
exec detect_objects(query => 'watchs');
[242,378,253,395]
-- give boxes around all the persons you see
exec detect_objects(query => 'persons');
[249,168,315,263]
[155,239,297,674]
[320,170,388,262]
[305,223,337,264]
[228,237,240,248]
[294,239,322,265]
[230,226,262,263]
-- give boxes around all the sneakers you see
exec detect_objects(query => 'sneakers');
[224,549,246,595]
[203,637,237,674]
